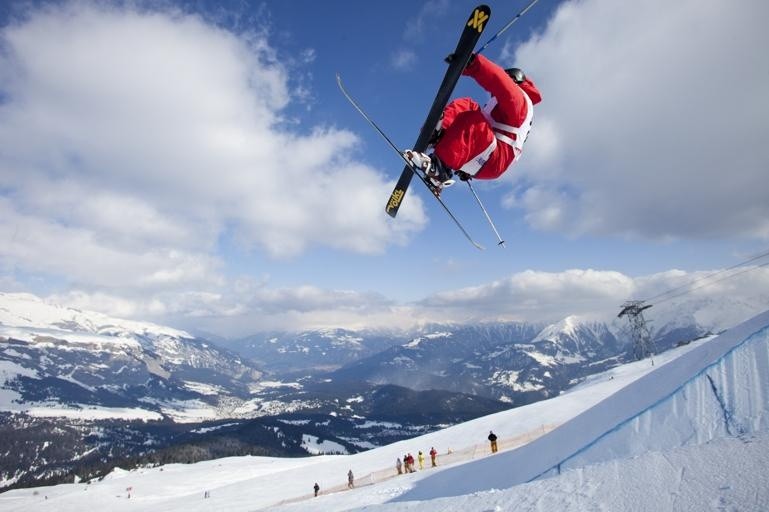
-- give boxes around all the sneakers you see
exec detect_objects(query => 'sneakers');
[420,111,444,147]
[404,149,451,187]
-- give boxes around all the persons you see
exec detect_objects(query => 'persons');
[403,50,542,195]
[488,430,498,452]
[348,469,354,488]
[403,454,409,472]
[396,456,403,473]
[418,450,425,469]
[430,446,437,466]
[314,482,319,496]
[407,453,416,472]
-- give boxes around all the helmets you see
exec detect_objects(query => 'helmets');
[505,68,525,83]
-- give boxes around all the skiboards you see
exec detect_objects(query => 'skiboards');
[335,5,490,250]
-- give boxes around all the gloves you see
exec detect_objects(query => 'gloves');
[454,170,471,182]
[443,53,472,68]
